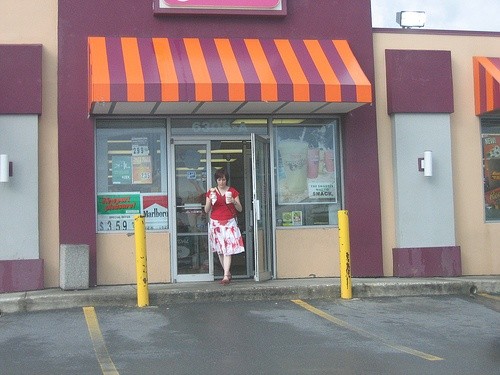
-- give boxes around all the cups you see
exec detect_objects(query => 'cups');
[279,138,334,195]
[225,191,232,204]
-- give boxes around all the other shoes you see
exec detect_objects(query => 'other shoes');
[220,275,229,284]
[229,274,232,282]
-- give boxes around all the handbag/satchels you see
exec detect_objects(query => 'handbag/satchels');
[236,221,243,233]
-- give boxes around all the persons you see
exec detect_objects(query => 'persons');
[204,169,242,285]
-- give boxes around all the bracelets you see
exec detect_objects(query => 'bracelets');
[232,198,237,205]
